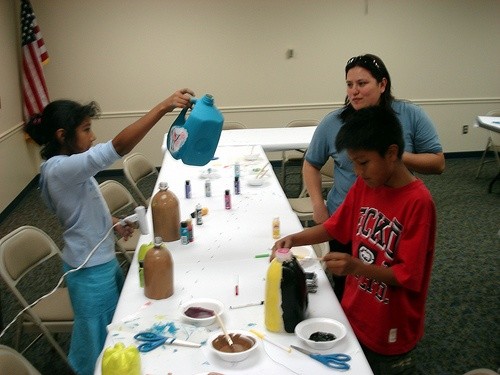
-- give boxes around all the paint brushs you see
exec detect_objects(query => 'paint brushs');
[213,310,237,352]
[256,161,269,178]
[259,169,269,178]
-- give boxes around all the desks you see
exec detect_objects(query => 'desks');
[161,126,317,152]
[473,116,500,182]
[94,145,373,375]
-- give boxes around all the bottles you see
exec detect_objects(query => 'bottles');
[224,189,232,210]
[151,181,181,242]
[234,161,240,176]
[166,93,225,167]
[204,180,211,197]
[264,247,309,334]
[272,216,280,239]
[138,235,174,300]
[196,203,203,225]
[186,219,193,242]
[235,176,240,195]
[180,221,189,245]
[185,180,191,198]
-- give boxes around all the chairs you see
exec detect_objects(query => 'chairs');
[122,153,159,211]
[0,345,41,375]
[99,180,141,267]
[0,225,81,375]
[282,118,500,375]
[221,121,247,130]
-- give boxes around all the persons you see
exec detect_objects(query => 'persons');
[23,88,195,375]
[269,105,437,375]
[302,53,446,302]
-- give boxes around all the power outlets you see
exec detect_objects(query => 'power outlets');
[463,125,469,134]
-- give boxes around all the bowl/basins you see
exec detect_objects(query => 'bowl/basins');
[247,173,271,185]
[295,317,348,350]
[180,298,225,327]
[209,330,259,363]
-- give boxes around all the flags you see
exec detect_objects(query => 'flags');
[19,1,50,142]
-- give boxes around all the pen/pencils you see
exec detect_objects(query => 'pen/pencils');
[255,253,272,258]
[293,255,322,260]
[250,329,290,352]
[235,275,239,295]
[230,301,264,309]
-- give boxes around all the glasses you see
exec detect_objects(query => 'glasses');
[346,55,385,76]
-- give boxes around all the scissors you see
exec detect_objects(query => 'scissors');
[291,345,351,370]
[135,332,202,353]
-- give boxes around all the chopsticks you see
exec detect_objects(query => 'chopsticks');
[256,161,271,179]
